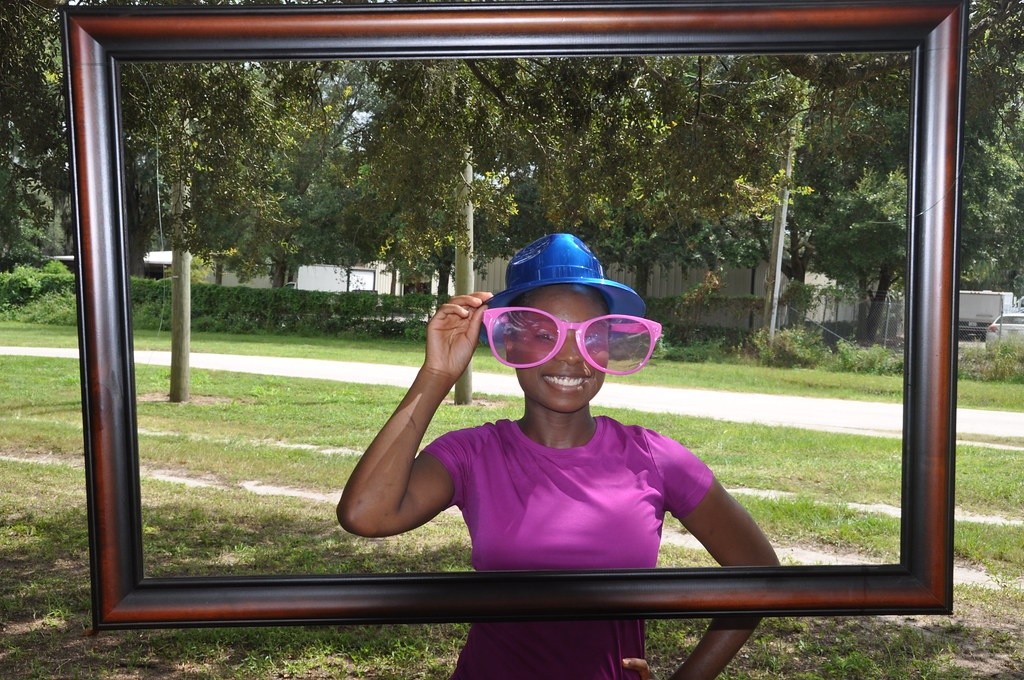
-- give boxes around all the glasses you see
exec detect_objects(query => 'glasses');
[483,306,663,375]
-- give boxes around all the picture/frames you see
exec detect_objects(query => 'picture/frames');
[59,0,968,629]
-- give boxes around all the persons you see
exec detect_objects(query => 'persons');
[336,232,780,680]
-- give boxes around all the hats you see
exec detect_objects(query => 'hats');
[476,233,645,345]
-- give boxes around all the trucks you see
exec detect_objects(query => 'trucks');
[958,289,1017,342]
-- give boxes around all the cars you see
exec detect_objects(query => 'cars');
[985,312,1024,352]
